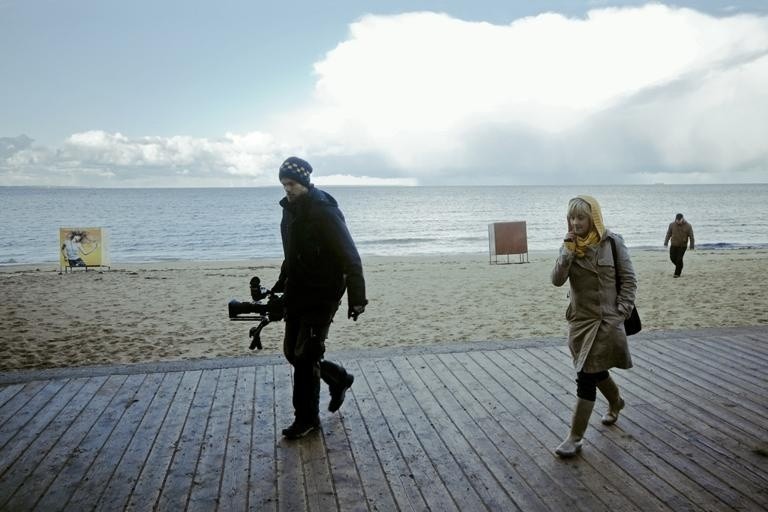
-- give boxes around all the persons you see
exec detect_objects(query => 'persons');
[551,194,636,459]
[61,229,98,267]
[664,213,695,277]
[270,157,368,438]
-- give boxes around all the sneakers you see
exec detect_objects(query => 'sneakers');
[329,375,354,412]
[283,418,319,438]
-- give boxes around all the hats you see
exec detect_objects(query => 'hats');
[279,157,311,186]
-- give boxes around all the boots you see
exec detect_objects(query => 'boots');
[555,397,595,457]
[595,374,624,425]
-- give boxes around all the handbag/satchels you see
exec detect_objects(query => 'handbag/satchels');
[624,305,641,336]
